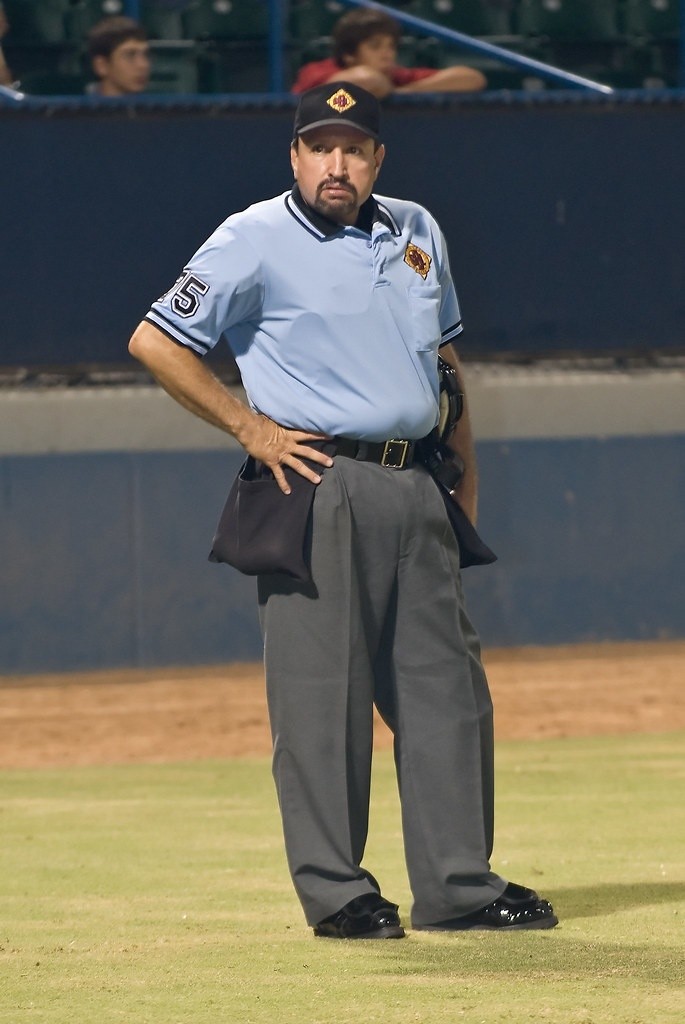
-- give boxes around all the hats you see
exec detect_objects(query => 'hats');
[294,80,381,140]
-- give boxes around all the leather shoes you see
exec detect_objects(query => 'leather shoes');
[412,882,559,931]
[314,892,405,938]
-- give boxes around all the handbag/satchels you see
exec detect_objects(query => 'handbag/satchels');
[423,461,499,568]
[207,445,337,580]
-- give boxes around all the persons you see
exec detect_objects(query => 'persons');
[0,13,485,97]
[127,82,560,939]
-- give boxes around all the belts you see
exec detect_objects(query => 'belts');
[320,435,426,471]
